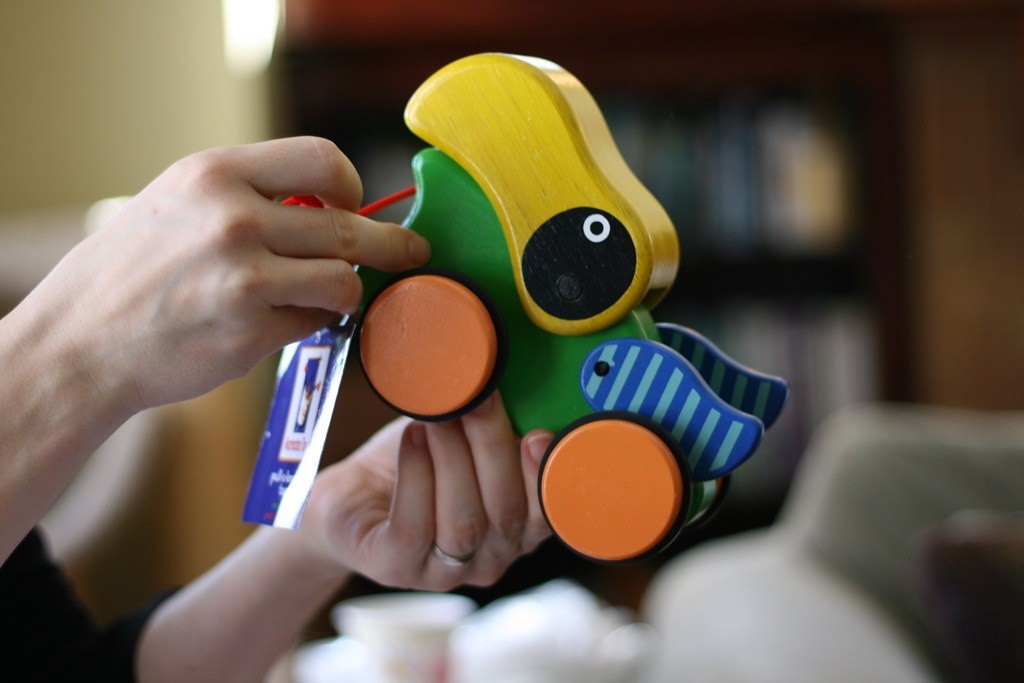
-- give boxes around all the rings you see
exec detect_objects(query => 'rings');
[430,543,476,566]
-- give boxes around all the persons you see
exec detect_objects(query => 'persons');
[0,135,551,683]
